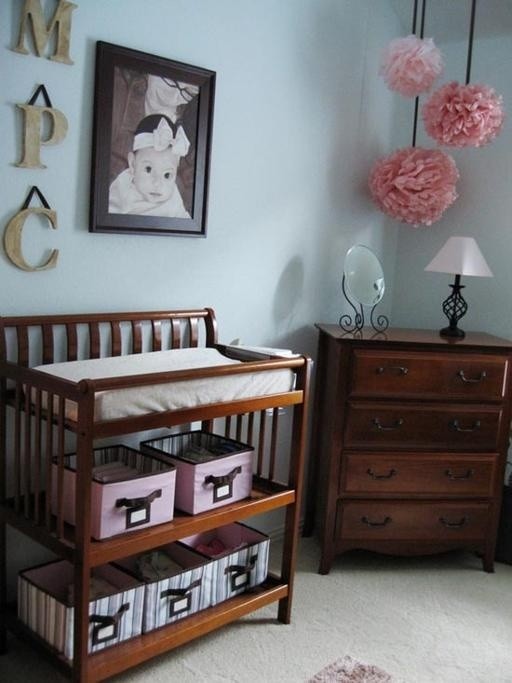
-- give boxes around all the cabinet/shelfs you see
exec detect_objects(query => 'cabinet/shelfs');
[302,324,512,575]
[0,308,314,683]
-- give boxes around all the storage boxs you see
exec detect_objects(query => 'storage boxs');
[112,541,213,635]
[140,430,255,515]
[176,520,271,607]
[48,444,178,540]
[17,556,146,660]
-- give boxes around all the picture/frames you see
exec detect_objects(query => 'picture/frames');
[88,40,216,237]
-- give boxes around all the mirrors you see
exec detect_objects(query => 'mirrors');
[339,244,388,334]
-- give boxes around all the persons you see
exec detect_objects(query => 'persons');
[107,111,193,220]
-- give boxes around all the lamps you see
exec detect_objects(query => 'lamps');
[423,236,494,338]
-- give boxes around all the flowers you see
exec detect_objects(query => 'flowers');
[379,0,447,97]
[366,0,460,227]
[423,0,504,148]
